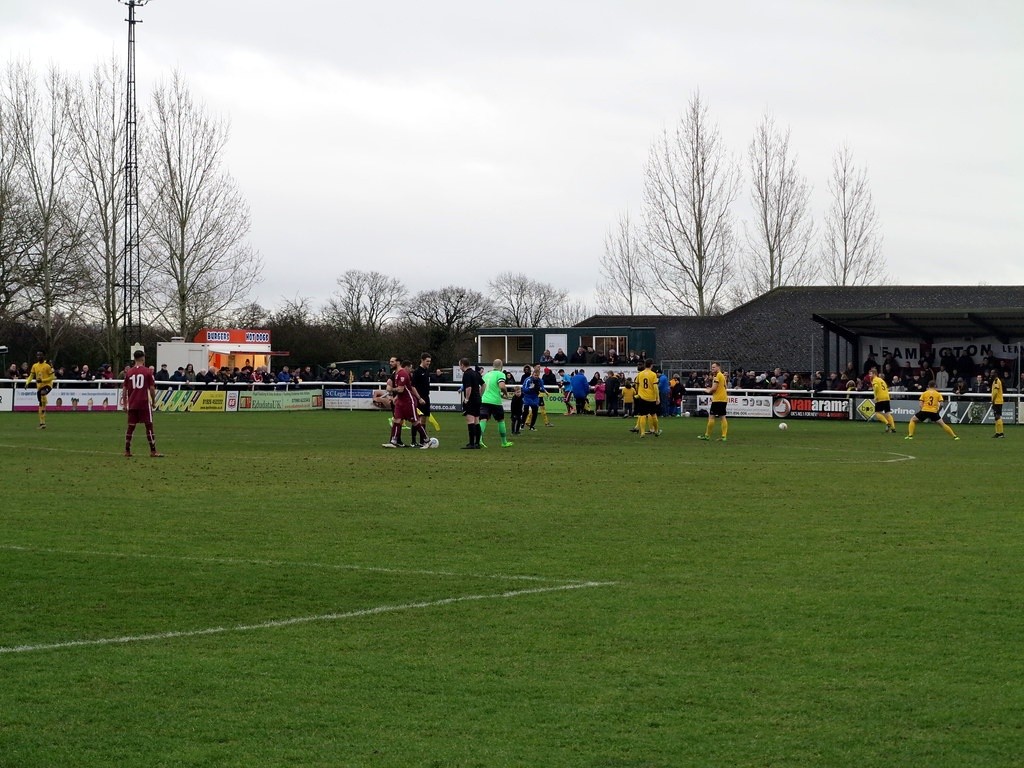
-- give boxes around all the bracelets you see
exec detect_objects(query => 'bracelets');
[465,399,468,401]
[419,397,421,400]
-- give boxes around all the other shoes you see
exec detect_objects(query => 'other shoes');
[645,430,655,434]
[886,423,896,433]
[629,428,639,433]
[151,451,163,457]
[39,424,46,430]
[526,423,535,427]
[640,436,645,439]
[655,429,662,438]
[511,432,522,437]
[546,423,554,427]
[529,426,537,432]
[520,423,524,430]
[125,450,132,457]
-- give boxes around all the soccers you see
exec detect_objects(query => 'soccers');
[778,422,787,430]
[685,411,690,417]
[429,437,439,448]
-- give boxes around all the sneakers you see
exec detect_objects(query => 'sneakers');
[461,442,480,449]
[479,441,487,448]
[420,441,432,449]
[992,433,1003,439]
[410,442,423,447]
[953,436,960,441]
[391,439,405,447]
[717,436,726,442]
[501,441,512,447]
[904,435,912,440]
[382,443,396,448]
[698,435,710,441]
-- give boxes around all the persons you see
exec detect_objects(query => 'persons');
[123,350,164,457]
[458,358,486,450]
[432,369,446,391]
[629,358,662,437]
[382,353,432,450]
[56,397,108,410]
[458,367,516,393]
[540,345,647,418]
[656,369,687,417]
[905,381,959,440]
[697,362,728,441]
[3,363,130,388]
[149,359,387,390]
[25,351,54,430]
[511,365,555,434]
[989,369,1004,438]
[685,350,1024,395]
[477,359,513,448]
[869,369,897,433]
